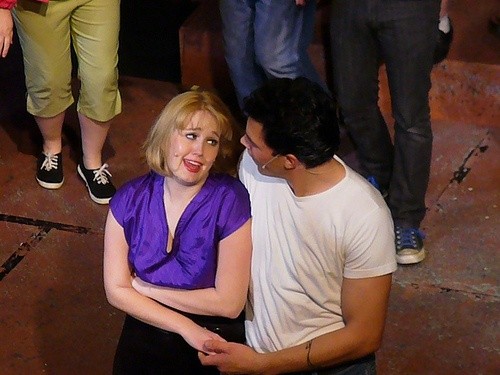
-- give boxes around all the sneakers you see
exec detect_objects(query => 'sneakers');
[394,225,426,265]
[77,157,116,204]
[432,16,453,62]
[36,148,63,189]
[363,172,388,197]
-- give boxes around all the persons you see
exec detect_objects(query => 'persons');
[321,0,446,264]
[198,76,398,375]
[103,88,251,375]
[0,0,122,205]
[219,0,335,116]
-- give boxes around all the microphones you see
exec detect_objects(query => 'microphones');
[170,144,178,157]
[262,154,281,170]
